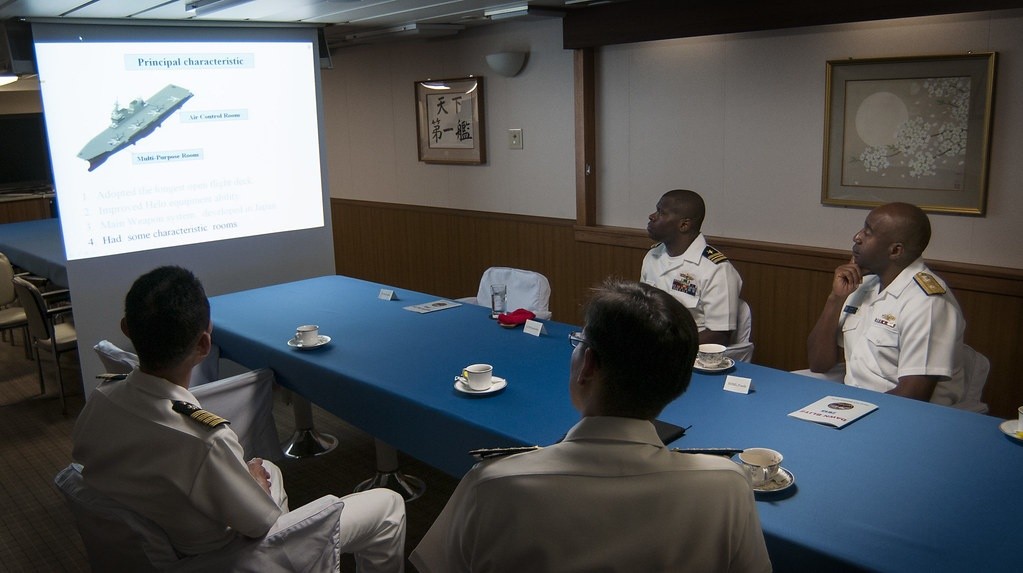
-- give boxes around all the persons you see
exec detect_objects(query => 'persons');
[406,273,772,573]
[71,265,405,573]
[639,189,743,347]
[806,202,967,408]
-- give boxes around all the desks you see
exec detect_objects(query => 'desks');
[208,275,1023,573]
[0,218,68,287]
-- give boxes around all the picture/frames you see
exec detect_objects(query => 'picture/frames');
[820,52,996,215]
[414,76,486,166]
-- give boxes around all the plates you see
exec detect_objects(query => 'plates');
[454,376,507,394]
[287,335,331,350]
[752,466,794,492]
[999,419,1023,441]
[692,357,735,373]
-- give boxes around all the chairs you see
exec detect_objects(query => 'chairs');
[94,340,282,466]
[0,252,83,416]
[790,343,989,413]
[53,462,344,573]
[453,267,553,321]
[724,296,754,365]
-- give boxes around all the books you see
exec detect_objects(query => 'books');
[652,419,685,446]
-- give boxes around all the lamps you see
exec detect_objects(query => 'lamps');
[485,52,526,76]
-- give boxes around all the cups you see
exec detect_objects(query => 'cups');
[739,448,783,487]
[462,364,493,390]
[295,325,319,346]
[490,284,507,319]
[697,344,726,368]
[1017,406,1023,431]
